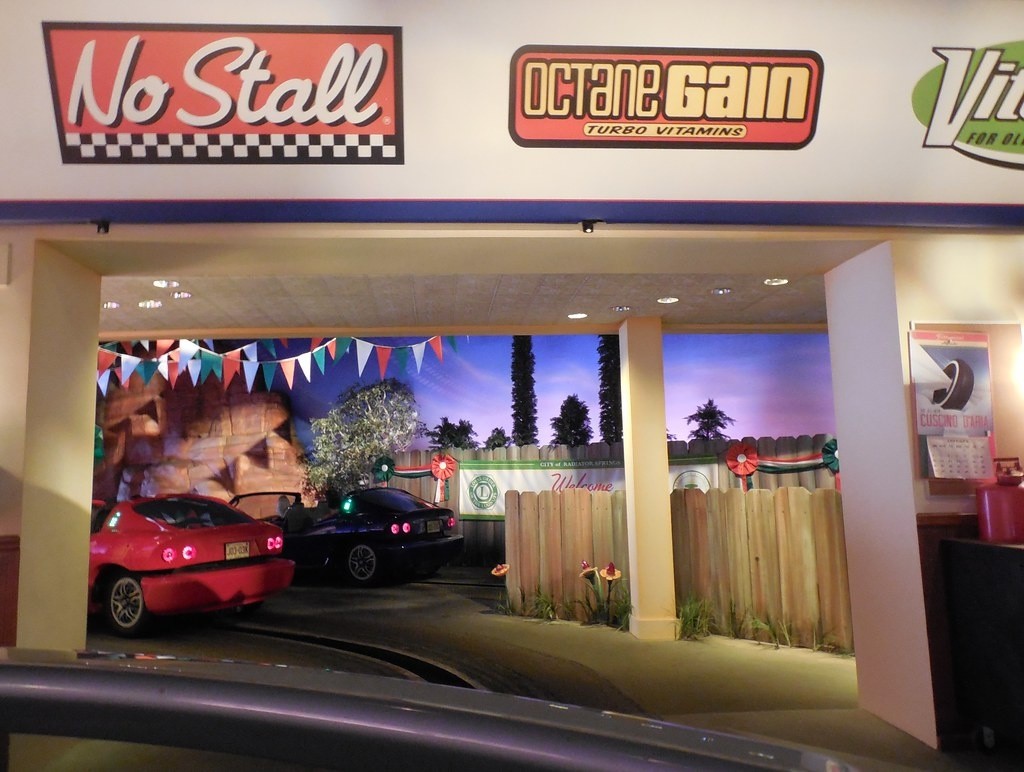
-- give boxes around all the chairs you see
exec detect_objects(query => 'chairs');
[311,501,331,524]
[283,503,309,534]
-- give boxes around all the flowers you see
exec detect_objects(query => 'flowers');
[491,560,622,625]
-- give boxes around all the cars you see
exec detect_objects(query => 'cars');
[88,492,297,639]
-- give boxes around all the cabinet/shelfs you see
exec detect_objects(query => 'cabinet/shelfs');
[939,535,1024,752]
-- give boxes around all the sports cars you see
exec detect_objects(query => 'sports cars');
[227,486,466,586]
